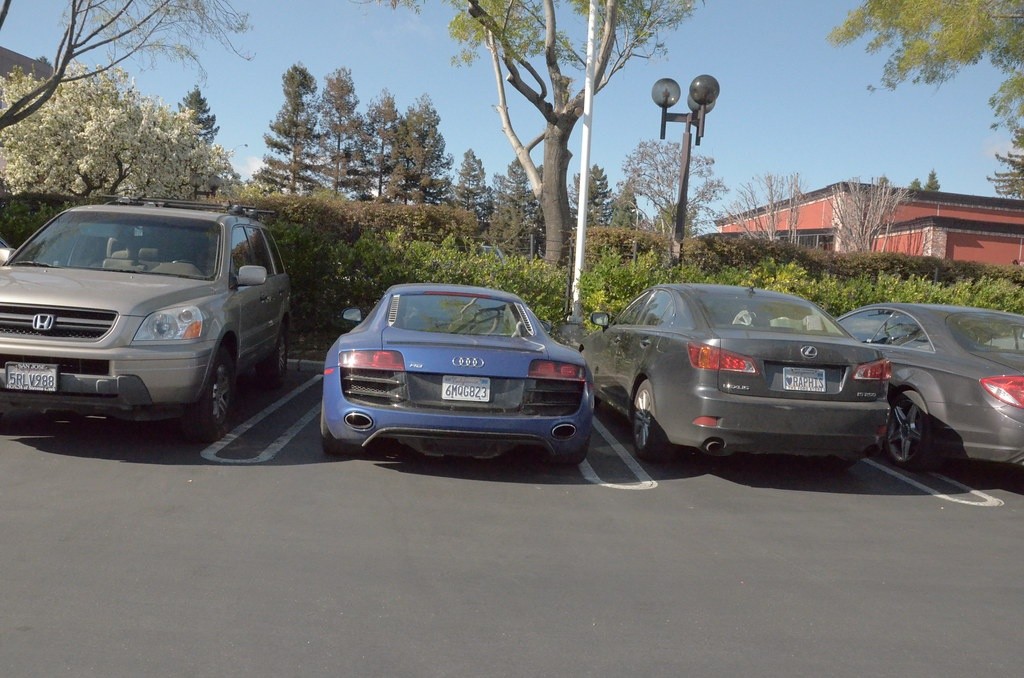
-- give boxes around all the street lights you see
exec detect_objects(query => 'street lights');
[650,74,720,287]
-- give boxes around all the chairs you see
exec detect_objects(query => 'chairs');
[101,236,215,276]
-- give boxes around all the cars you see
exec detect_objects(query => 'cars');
[835,305,1023,475]
[578,282,891,471]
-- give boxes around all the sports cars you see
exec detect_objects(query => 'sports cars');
[320,281,594,473]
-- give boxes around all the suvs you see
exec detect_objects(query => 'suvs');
[0,193,290,445]
